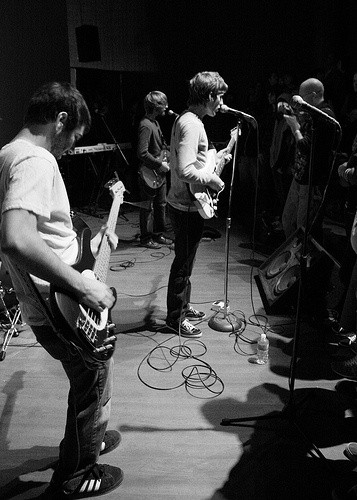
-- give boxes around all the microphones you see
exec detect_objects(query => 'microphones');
[95,109,104,116]
[168,110,180,118]
[221,105,255,120]
[290,95,341,127]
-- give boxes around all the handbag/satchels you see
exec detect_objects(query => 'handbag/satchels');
[248,216,284,254]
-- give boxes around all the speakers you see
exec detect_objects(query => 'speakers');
[257,225,343,314]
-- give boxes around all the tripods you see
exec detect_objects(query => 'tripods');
[222,117,331,462]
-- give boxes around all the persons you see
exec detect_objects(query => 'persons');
[84,85,152,211]
[137,89,176,249]
[210,50,357,236]
[322,154,357,434]
[0,78,126,500]
[165,70,242,338]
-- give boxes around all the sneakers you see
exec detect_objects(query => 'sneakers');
[165,318,202,338]
[330,356,356,377]
[99,430,121,456]
[141,241,162,249]
[157,237,172,244]
[47,464,124,499]
[186,303,206,321]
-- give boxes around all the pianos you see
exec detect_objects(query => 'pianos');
[62,143,133,155]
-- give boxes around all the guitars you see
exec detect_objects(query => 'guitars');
[190,127,242,219]
[52,179,126,360]
[142,150,172,189]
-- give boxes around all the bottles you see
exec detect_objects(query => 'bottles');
[256,334,269,365]
